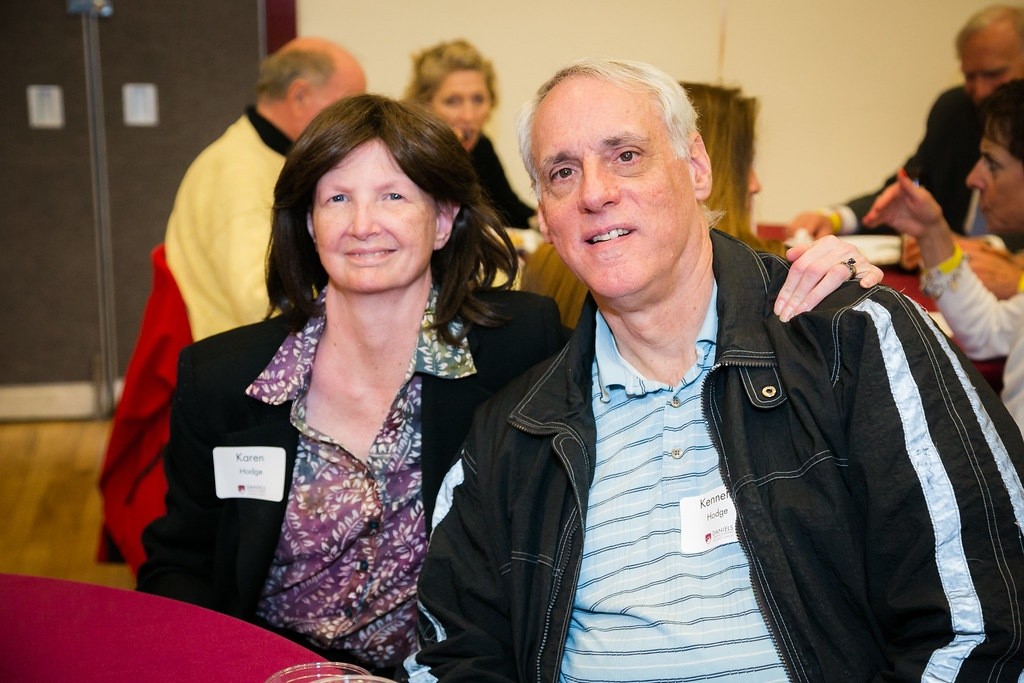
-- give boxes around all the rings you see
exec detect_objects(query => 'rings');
[841,258,857,281]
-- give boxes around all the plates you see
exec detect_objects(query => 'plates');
[841,235,901,266]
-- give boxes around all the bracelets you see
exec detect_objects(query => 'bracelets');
[921,244,967,297]
[820,211,840,234]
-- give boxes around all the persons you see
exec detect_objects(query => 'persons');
[136,96,883,683]
[405,39,539,232]
[864,77,1024,438]
[165,38,368,340]
[519,83,770,328]
[787,5,1024,241]
[404,56,1024,683]
